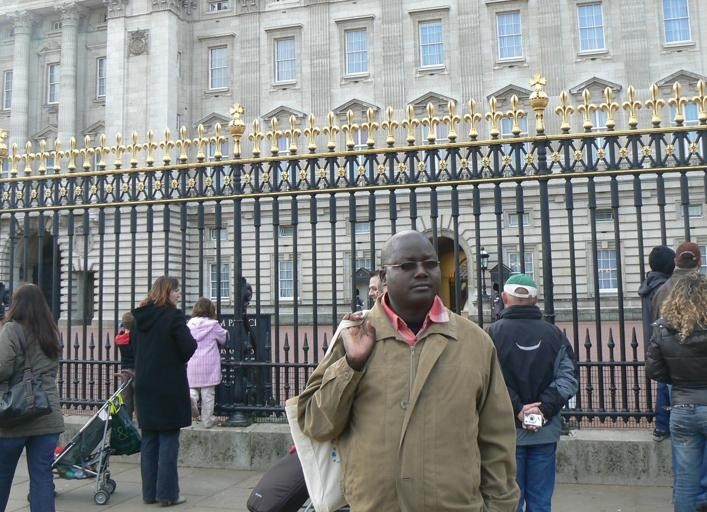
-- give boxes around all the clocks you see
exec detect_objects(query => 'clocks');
[130,38,145,54]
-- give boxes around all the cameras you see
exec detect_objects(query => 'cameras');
[524,414,542,430]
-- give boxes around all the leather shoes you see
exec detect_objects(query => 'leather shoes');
[161,495,186,506]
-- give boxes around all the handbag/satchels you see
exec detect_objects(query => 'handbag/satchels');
[0,377,52,429]
[107,405,141,455]
[284,396,348,511]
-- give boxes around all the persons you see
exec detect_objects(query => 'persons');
[375,277,388,297]
[133,276,198,506]
[368,271,380,304]
[0,283,63,512]
[485,272,578,512]
[116,313,141,428]
[637,246,676,442]
[652,241,707,512]
[185,297,227,429]
[644,270,707,512]
[298,230,520,512]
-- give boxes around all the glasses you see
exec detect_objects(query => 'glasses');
[382,261,441,271]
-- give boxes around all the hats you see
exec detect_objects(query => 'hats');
[502,272,539,300]
[649,245,676,274]
[672,243,702,267]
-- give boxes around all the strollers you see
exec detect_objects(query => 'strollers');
[51,369,134,505]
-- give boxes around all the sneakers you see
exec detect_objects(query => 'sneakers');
[203,415,218,428]
[651,428,670,442]
[190,397,199,418]
